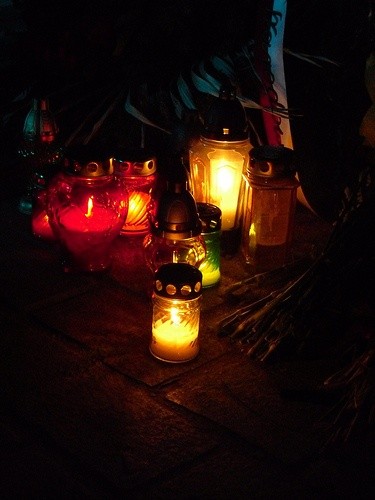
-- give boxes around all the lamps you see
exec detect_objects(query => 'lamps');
[194,84,254,253]
[119,153,161,236]
[47,154,128,271]
[16,99,63,214]
[188,202,222,288]
[149,262,201,363]
[147,170,206,276]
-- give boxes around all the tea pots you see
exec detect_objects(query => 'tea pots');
[35,160,131,267]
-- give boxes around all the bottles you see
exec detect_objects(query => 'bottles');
[195,201,223,288]
[13,91,66,217]
[150,261,202,361]
[242,145,300,245]
[149,176,204,271]
[188,83,253,235]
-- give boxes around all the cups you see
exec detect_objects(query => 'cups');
[105,150,160,237]
